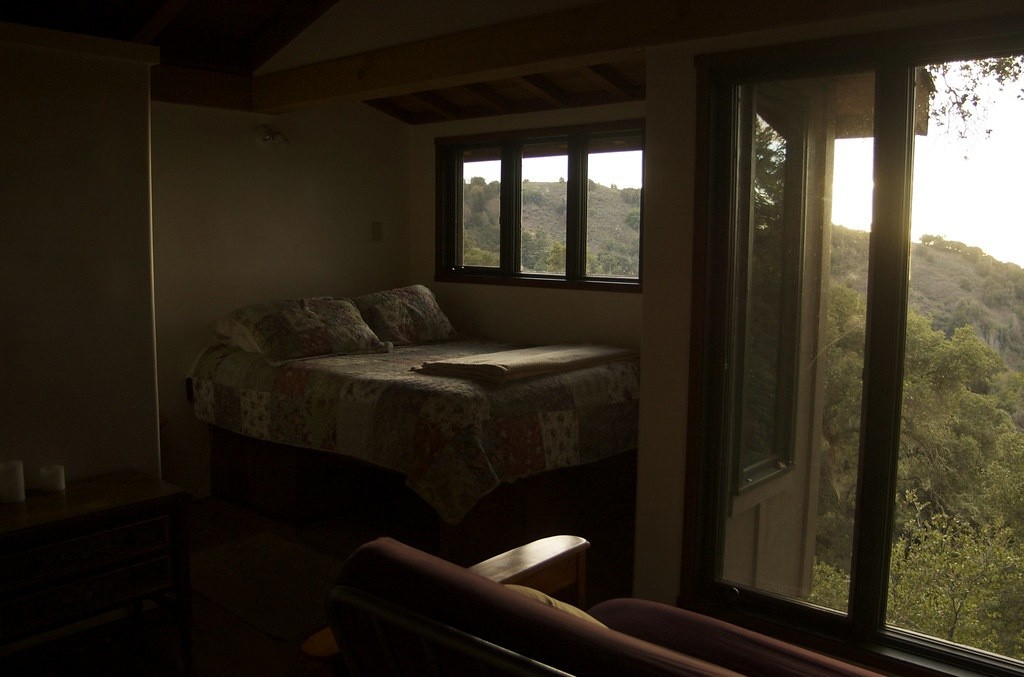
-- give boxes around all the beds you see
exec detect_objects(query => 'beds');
[185,336,639,596]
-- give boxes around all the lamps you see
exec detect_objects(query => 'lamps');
[256,124,290,145]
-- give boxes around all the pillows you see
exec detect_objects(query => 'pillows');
[353,284,464,349]
[226,298,395,368]
[216,316,258,353]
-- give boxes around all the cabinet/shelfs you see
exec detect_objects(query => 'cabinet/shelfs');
[0,470,197,677]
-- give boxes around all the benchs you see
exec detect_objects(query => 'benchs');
[328,533,897,677]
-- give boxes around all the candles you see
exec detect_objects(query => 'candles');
[0,460,26,503]
[40,464,65,491]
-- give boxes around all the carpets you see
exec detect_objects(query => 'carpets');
[187,532,342,639]
